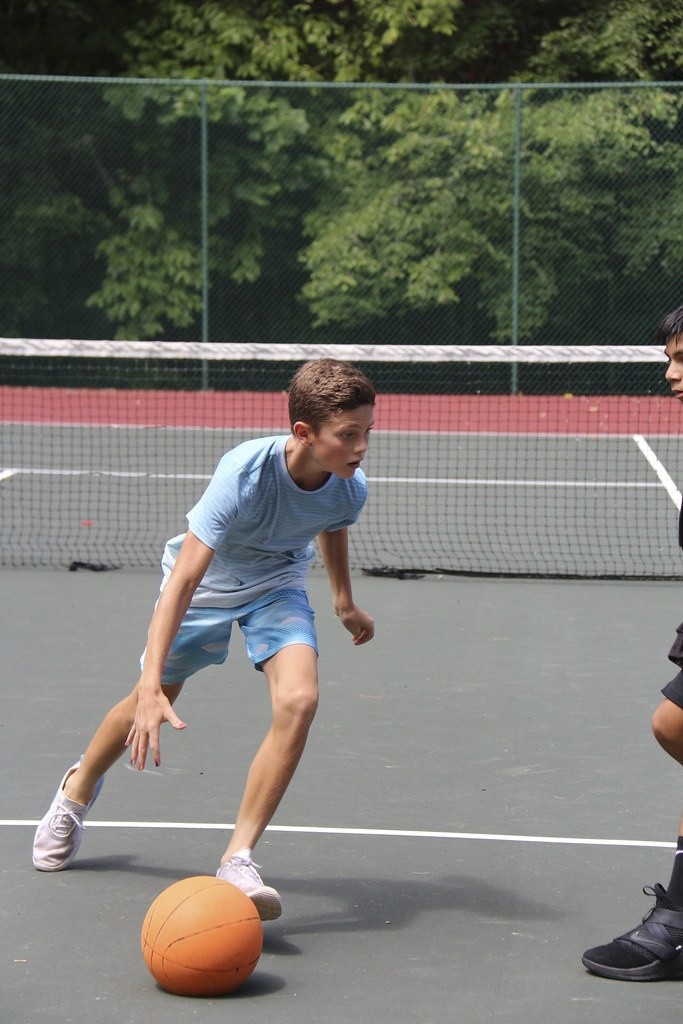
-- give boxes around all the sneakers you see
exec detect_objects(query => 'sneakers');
[32,761,105,871]
[217,848,282,921]
[580,882,683,980]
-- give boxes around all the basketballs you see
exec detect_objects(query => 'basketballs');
[141,876,263,997]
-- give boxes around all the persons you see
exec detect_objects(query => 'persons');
[580,302,683,1001]
[33,352,382,923]
